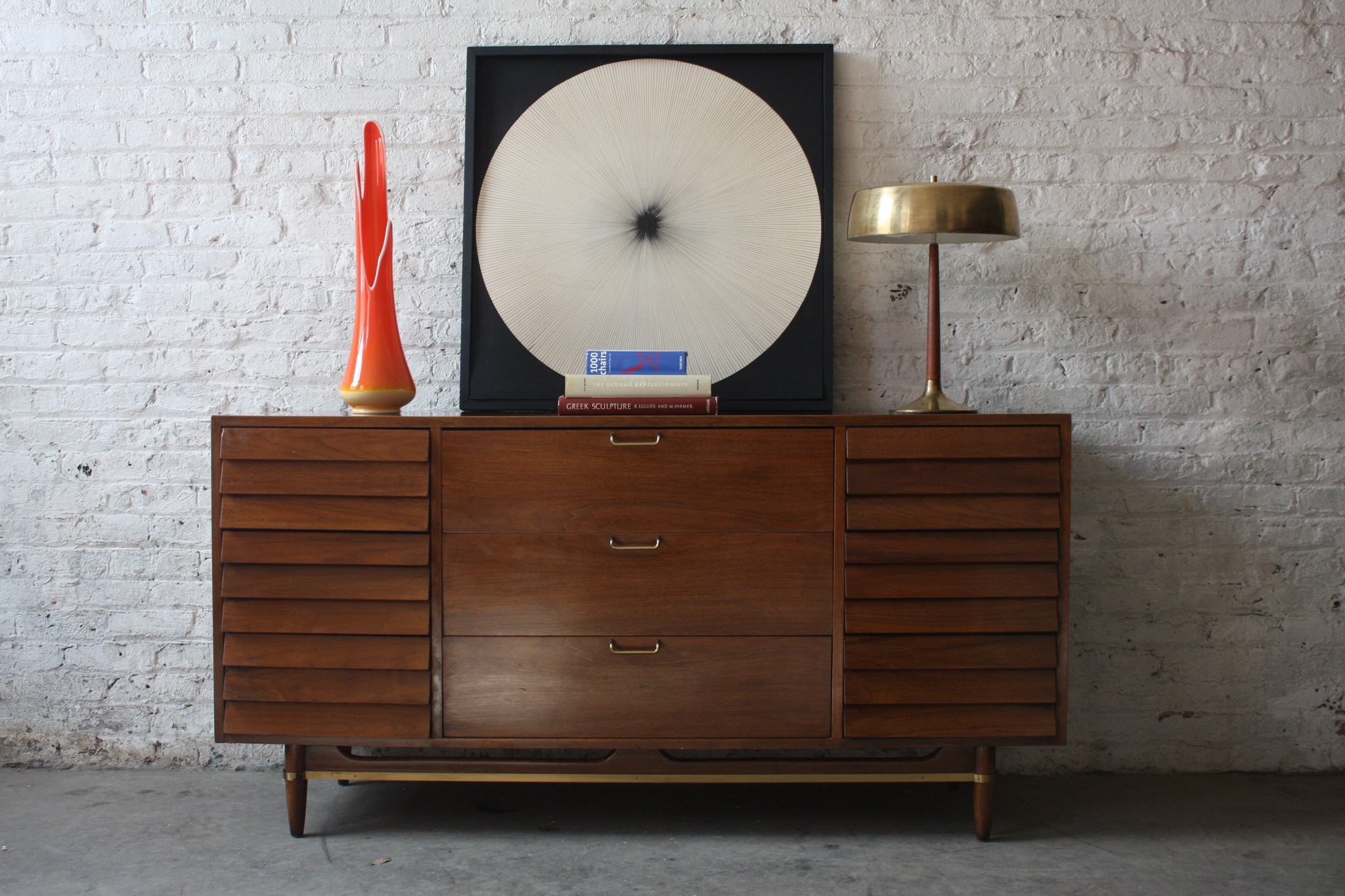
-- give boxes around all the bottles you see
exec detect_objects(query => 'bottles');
[340,120,416,413]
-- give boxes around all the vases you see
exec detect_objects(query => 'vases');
[340,119,418,415]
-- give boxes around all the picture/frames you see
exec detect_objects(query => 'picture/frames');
[459,43,833,414]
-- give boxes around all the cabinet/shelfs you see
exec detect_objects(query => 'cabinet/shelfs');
[211,413,1072,842]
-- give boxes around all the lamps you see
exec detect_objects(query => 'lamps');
[847,174,1021,412]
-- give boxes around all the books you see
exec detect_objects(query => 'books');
[558,350,718,415]
